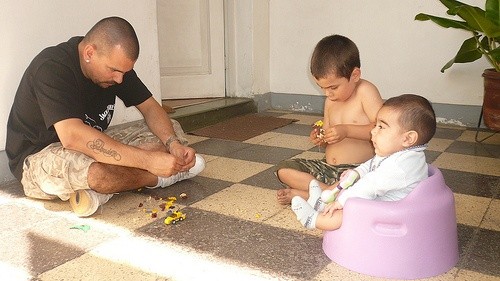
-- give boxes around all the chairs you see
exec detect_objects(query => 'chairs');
[323,166,459,279]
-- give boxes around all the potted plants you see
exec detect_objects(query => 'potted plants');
[414,0,500,142]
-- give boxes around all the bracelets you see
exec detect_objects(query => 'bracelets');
[165,135,178,152]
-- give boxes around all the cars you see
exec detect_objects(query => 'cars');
[164,211,186,225]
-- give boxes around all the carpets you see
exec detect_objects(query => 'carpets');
[187,113,300,142]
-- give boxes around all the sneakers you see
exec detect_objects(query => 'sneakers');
[291,195,317,230]
[306,179,326,213]
[69,189,119,216]
[161,153,206,188]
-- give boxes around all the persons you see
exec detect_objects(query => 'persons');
[274,34,383,205]
[292,94,436,231]
[6,16,206,218]
